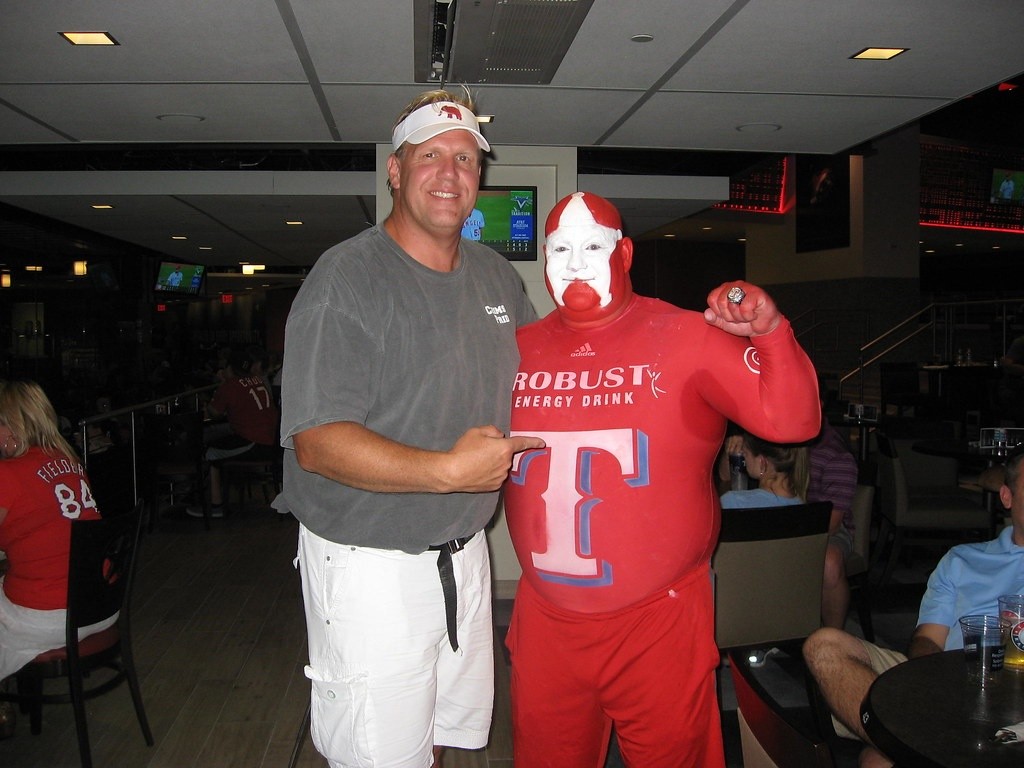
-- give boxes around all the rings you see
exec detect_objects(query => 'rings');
[726,287,745,305]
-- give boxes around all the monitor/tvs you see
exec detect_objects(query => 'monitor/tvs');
[155,261,205,295]
[989,168,1024,208]
[459,186,537,261]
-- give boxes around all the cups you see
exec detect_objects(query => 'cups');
[728,450,748,490]
[997,595,1024,673]
[993,427,1008,449]
[959,615,1013,688]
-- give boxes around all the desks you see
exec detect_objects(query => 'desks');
[912,438,1015,541]
[830,414,880,464]
[860,649,1024,768]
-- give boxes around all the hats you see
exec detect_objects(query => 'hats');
[227,350,253,376]
[392,101,490,153]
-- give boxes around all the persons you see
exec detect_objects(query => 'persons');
[802,444,1024,768]
[0,339,281,748]
[998,334,1023,428]
[281,93,546,767]
[713,414,859,630]
[499,191,823,768]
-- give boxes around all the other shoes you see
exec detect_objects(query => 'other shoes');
[748,646,780,667]
[0,705,17,737]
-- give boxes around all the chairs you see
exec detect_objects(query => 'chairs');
[711,501,836,760]
[845,482,877,644]
[726,648,835,768]
[868,433,995,608]
[879,416,958,569]
[0,498,154,768]
[222,392,285,523]
[879,362,932,439]
[143,410,210,534]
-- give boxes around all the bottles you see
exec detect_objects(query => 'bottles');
[952,347,963,365]
[964,348,972,364]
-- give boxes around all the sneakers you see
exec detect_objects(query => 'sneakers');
[186,503,226,517]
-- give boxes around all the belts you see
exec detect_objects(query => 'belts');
[428,533,476,656]
